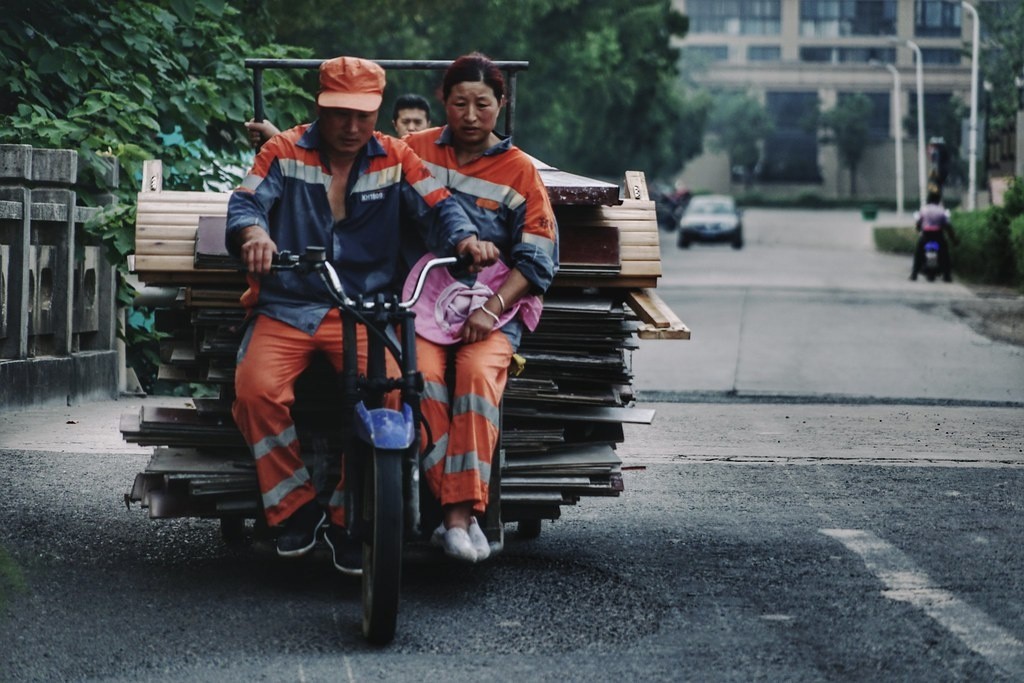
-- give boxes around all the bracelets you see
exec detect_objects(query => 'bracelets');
[494,292,506,313]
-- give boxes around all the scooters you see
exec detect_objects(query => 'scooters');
[913,221,947,283]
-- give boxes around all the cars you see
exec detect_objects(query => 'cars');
[678,194,746,250]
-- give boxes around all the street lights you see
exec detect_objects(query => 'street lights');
[888,36,929,207]
[867,56,907,227]
[947,0,981,212]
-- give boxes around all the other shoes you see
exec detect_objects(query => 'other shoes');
[943,276,952,282]
[324,523,363,576]
[430,516,491,563]
[276,498,327,556]
[909,276,917,280]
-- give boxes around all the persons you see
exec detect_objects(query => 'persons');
[909,166,955,284]
[244,54,558,562]
[224,58,500,573]
[392,94,431,139]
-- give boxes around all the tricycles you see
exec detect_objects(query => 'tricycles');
[212,239,546,647]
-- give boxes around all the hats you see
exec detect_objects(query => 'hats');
[402,250,522,346]
[317,55,386,113]
